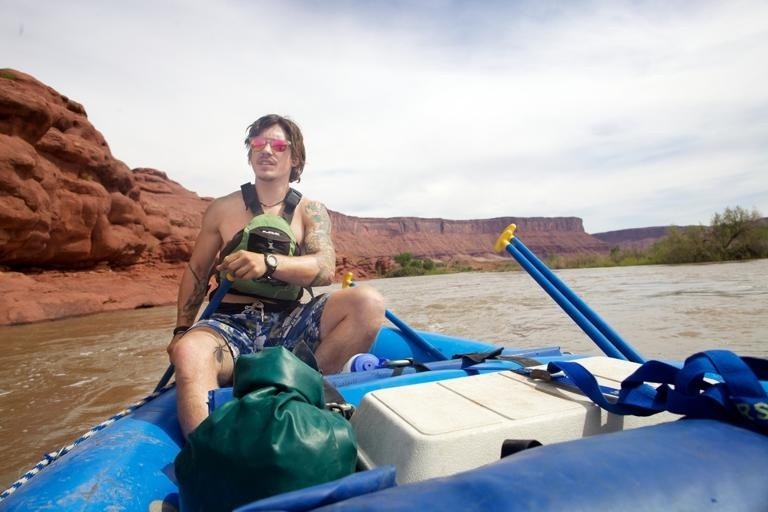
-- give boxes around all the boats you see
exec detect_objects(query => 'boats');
[1,321,768,512]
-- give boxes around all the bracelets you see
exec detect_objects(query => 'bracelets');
[174,326,191,336]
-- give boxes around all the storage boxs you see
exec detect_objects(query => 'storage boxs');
[346,356,676,489]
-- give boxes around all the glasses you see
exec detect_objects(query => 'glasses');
[245,134,293,154]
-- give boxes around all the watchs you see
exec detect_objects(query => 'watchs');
[261,251,278,281]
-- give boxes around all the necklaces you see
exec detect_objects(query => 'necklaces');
[259,198,286,207]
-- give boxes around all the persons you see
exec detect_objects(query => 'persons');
[168,113,386,446]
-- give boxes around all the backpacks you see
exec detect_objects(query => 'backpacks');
[215,182,305,304]
